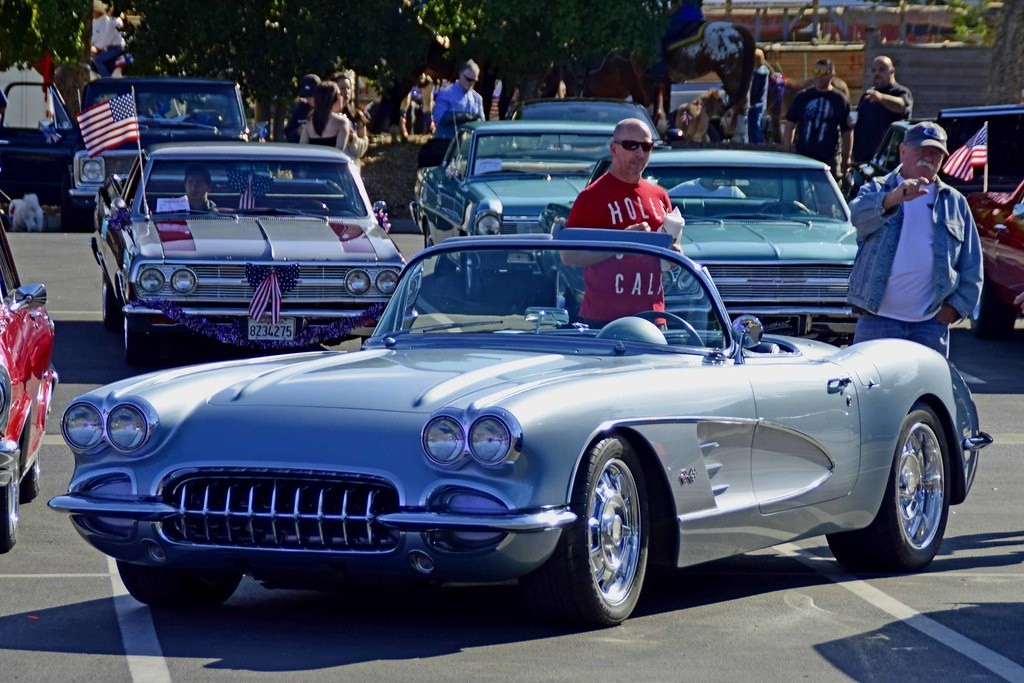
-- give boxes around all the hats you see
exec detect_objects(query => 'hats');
[298,75,322,98]
[904,121,950,156]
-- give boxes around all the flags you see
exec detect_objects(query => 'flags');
[76,90,139,159]
[942,125,989,181]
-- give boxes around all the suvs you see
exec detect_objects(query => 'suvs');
[841,116,935,202]
[0,75,249,233]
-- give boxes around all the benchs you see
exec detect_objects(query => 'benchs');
[671,196,782,218]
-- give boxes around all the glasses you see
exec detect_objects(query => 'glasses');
[462,73,478,84]
[872,66,893,74]
[613,139,654,153]
[813,68,832,76]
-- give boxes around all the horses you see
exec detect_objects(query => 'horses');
[584,20,755,145]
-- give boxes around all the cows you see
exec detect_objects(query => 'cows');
[765,61,851,145]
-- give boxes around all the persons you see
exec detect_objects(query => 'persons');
[91,0,126,77]
[748,49,914,198]
[645,0,706,88]
[183,165,219,214]
[561,119,684,331]
[286,60,486,170]
[847,121,984,360]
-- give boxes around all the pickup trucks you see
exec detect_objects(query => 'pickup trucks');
[87,140,406,365]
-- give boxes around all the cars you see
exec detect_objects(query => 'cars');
[0,219,59,553]
[409,121,616,303]
[507,98,684,151]
[539,146,860,345]
[47,215,994,626]
[966,183,1024,339]
